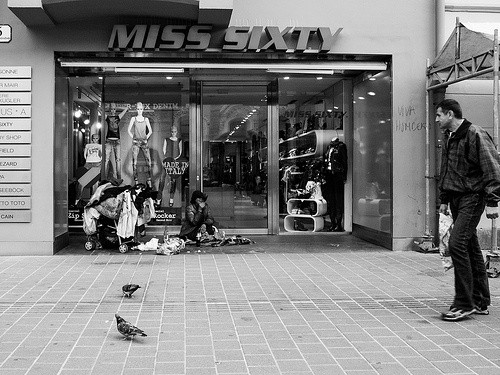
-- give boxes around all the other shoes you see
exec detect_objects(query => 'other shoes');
[333,226,341,232]
[155,200,162,208]
[327,225,335,231]
[146,179,152,189]
[170,200,173,207]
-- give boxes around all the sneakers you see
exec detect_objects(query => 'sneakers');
[471,304,490,315]
[441,307,476,320]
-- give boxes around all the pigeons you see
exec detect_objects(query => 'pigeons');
[122,284,141,297]
[115,313,147,340]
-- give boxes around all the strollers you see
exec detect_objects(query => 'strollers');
[82,180,158,253]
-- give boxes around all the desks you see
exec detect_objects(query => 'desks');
[186,185,234,220]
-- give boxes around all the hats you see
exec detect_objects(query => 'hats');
[190,190,208,204]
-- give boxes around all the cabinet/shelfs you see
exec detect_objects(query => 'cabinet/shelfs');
[258,127,344,232]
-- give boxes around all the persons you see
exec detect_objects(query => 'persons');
[179,190,217,247]
[436,99,500,322]
[98,103,131,180]
[324,136,349,232]
[156,127,183,207]
[127,102,153,188]
[75,135,102,204]
